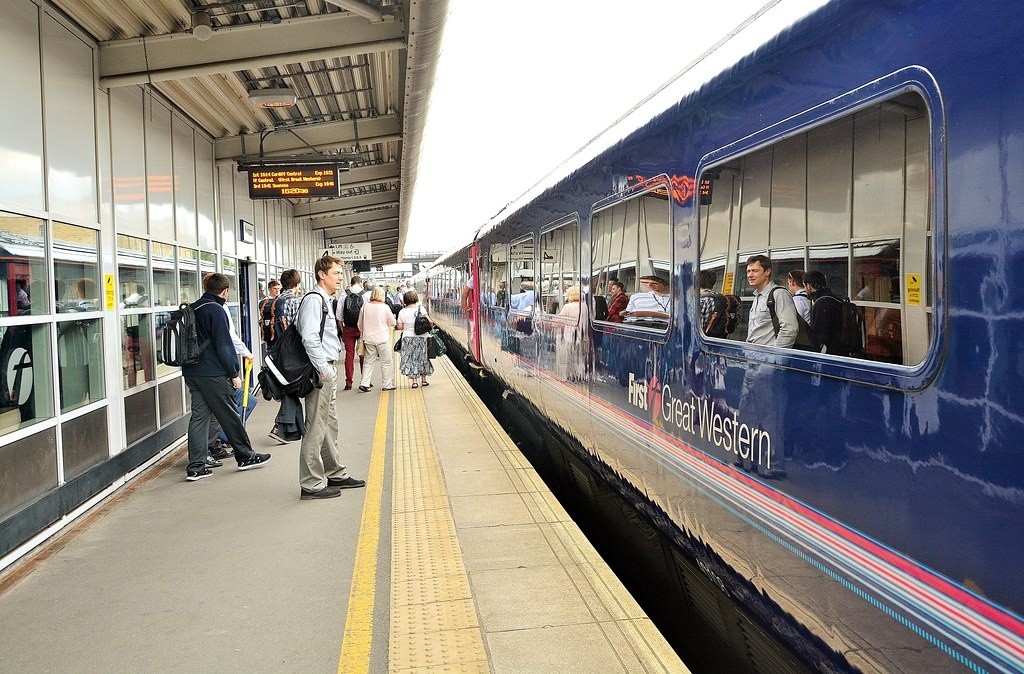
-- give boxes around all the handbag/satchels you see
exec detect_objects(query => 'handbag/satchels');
[427,334,447,359]
[394,332,403,353]
[393,304,401,315]
[768,287,827,354]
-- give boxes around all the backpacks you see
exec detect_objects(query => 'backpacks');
[343,288,367,327]
[257,291,329,402]
[161,301,227,366]
[700,294,742,337]
[259,294,295,344]
[414,304,432,335]
[813,295,869,358]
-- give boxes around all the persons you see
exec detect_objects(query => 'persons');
[444,256,900,481]
[460,274,474,311]
[161,256,434,500]
[0,277,151,423]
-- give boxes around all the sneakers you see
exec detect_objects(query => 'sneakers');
[185,470,213,481]
[237,453,272,472]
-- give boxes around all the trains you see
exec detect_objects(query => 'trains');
[397,0,1023,674]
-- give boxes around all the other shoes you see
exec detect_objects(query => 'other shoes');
[359,386,372,392]
[206,451,223,466]
[412,381,429,388]
[209,439,234,459]
[382,387,396,391]
[300,475,366,500]
[268,424,302,444]
